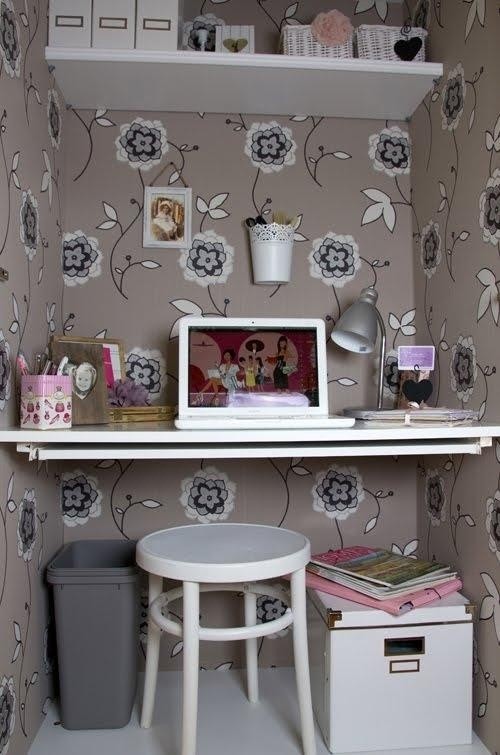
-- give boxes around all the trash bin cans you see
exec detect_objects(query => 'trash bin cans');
[46,539,142,731]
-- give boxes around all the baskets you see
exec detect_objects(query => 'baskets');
[353,24,428,62]
[278,24,355,58]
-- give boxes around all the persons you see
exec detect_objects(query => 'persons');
[191,336,290,407]
[154,200,181,241]
[75,362,96,391]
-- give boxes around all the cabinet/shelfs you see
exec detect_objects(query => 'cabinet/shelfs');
[0,48,500,465]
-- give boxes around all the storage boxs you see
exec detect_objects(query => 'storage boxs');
[308,588,480,755]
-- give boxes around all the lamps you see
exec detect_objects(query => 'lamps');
[329,285,396,422]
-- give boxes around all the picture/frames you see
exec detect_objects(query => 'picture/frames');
[139,184,195,252]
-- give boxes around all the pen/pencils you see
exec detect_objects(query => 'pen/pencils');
[17,353,69,375]
[273,208,299,227]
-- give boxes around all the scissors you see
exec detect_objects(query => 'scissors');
[246,215,267,228]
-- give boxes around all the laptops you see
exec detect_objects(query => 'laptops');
[173,317,356,430]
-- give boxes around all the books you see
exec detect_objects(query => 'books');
[306,546,456,601]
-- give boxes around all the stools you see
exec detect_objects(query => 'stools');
[132,519,321,755]
